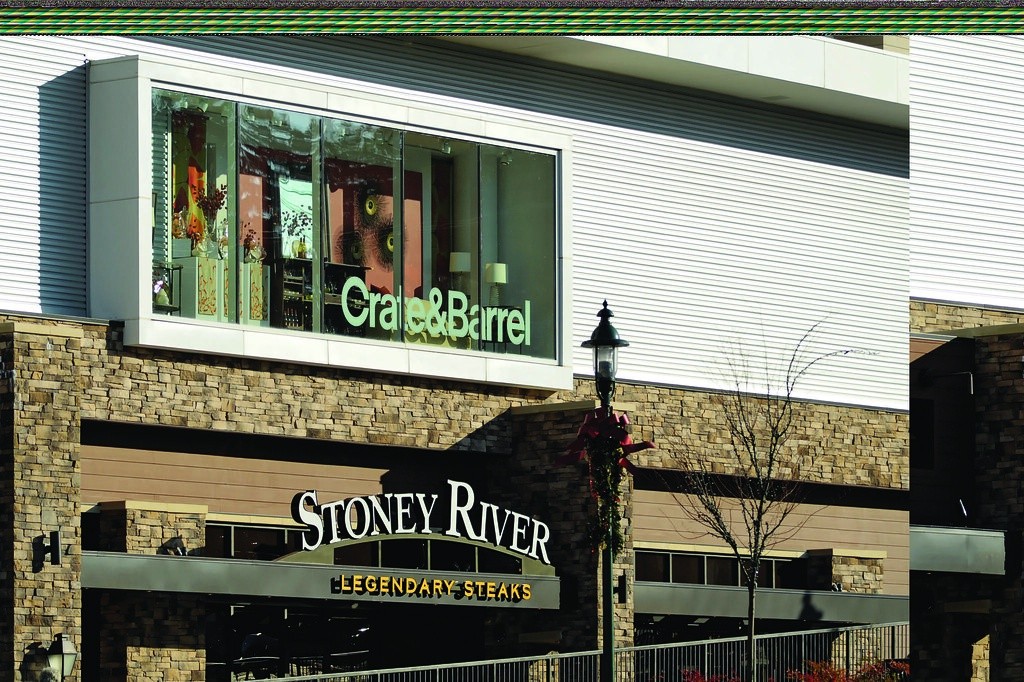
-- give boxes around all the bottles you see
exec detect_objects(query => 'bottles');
[298,237,307,259]
[179,205,189,230]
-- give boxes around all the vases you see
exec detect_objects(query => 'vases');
[156,288,169,305]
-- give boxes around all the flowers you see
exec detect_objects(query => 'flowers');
[244,222,261,251]
[152,279,169,294]
[191,180,225,236]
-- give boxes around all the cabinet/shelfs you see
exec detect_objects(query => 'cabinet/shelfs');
[269,257,371,339]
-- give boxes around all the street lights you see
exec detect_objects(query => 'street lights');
[579,298,632,682]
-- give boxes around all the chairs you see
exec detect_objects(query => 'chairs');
[482,305,524,352]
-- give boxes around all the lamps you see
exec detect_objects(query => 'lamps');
[613,575,626,604]
[48,633,78,682]
[485,262,510,306]
[440,144,451,153]
[44,531,62,564]
[448,251,471,296]
[196,102,208,113]
[500,155,511,165]
